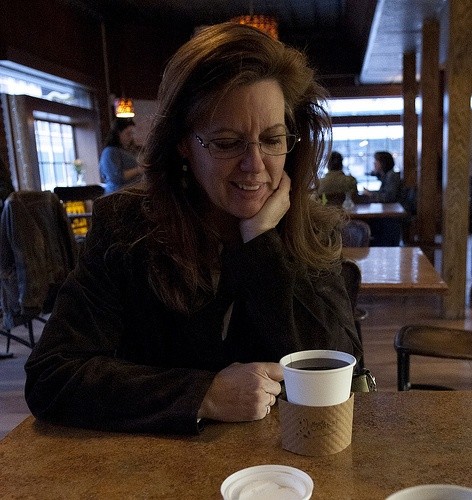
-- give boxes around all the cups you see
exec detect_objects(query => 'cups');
[382,483,472,500]
[279,351,358,407]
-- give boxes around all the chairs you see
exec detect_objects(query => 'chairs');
[394,324,471,391]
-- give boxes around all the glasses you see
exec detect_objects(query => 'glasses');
[188,121,297,160]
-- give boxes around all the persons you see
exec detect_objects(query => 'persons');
[316,152,360,197]
[23,22,364,437]
[98,120,147,198]
[362,151,404,204]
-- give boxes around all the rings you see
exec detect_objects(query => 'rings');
[268,394,274,406]
[266,405,271,415]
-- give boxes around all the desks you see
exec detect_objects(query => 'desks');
[0,391,472,500]
[313,187,407,224]
[340,246,451,375]
